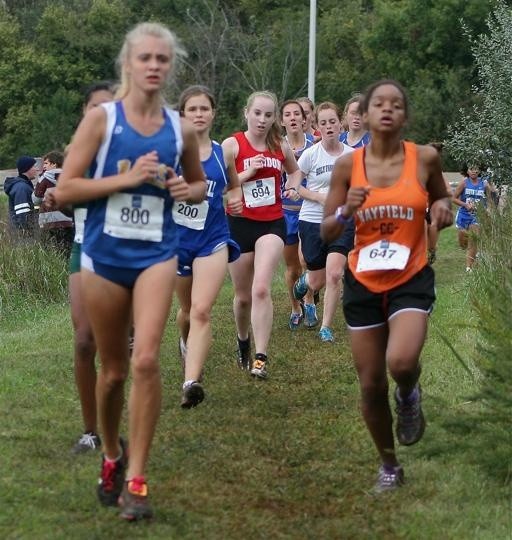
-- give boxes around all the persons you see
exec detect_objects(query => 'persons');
[221,90,370,378]
[426,214,438,264]
[34,150,75,258]
[451,158,492,271]
[320,80,452,489]
[4,157,41,237]
[175,85,244,409]
[54,21,206,521]
[482,166,511,215]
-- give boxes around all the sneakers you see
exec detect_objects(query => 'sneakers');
[251,353,268,379]
[293,272,309,301]
[71,431,101,454]
[318,326,334,343]
[377,463,404,491]
[304,303,320,330]
[288,302,304,331]
[97,437,128,506]
[394,383,425,447]
[117,477,154,521]
[237,332,253,371]
[181,380,205,409]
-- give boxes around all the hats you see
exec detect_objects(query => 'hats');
[16,157,36,173]
